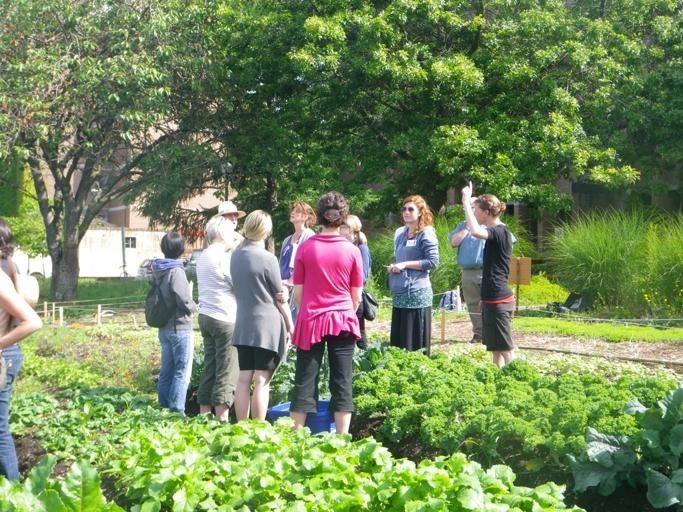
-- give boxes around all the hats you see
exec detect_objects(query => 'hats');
[215,201,247,220]
[6,256,40,334]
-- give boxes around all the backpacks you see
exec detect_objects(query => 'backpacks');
[145,274,177,328]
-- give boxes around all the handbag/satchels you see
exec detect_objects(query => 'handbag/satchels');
[363,292,378,320]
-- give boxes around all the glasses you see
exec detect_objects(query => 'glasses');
[402,206,415,212]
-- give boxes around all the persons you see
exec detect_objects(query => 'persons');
[0,218,20,291]
[196,201,295,423]
[277,191,369,433]
[450,196,517,343]
[0,268,42,481]
[151,231,197,417]
[462,181,515,370]
[386,196,440,357]
